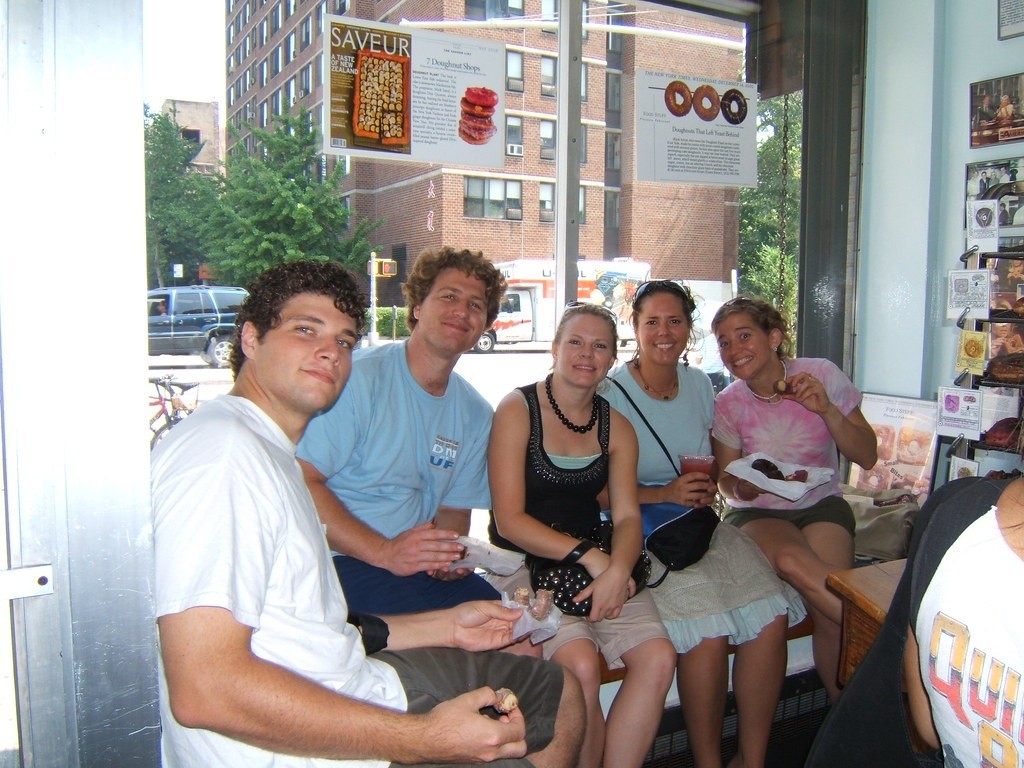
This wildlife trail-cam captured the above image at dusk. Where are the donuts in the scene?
[856,425,931,489]
[459,86,498,145]
[493,687,518,714]
[511,587,554,622]
[664,81,748,125]
[751,459,808,481]
[976,208,993,227]
[965,338,984,358]
[984,296,1024,446]
[773,380,797,395]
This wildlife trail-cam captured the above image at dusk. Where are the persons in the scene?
[158,300,166,316]
[710,297,878,702]
[801,473,1024,768]
[295,247,542,661]
[487,302,677,768]
[149,259,588,768]
[975,94,1024,359]
[596,280,808,768]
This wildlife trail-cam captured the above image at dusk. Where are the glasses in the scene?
[720,296,752,308]
[632,280,689,303]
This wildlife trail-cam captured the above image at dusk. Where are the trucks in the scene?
[471,257,652,355]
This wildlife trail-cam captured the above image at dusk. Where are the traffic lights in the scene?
[377,260,397,278]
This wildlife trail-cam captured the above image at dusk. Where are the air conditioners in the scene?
[508,143,524,158]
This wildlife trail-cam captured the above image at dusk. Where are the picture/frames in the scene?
[964,71,1024,458]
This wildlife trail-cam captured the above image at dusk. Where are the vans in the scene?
[147,284,256,369]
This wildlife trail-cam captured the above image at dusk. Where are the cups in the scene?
[679,453,715,503]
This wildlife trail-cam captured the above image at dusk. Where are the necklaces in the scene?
[751,360,787,399]
[546,374,598,433]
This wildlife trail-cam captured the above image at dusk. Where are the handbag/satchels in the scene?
[530,520,652,615]
[803,629,922,768]
[640,502,720,588]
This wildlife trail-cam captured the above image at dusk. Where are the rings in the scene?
[808,373,811,379]
